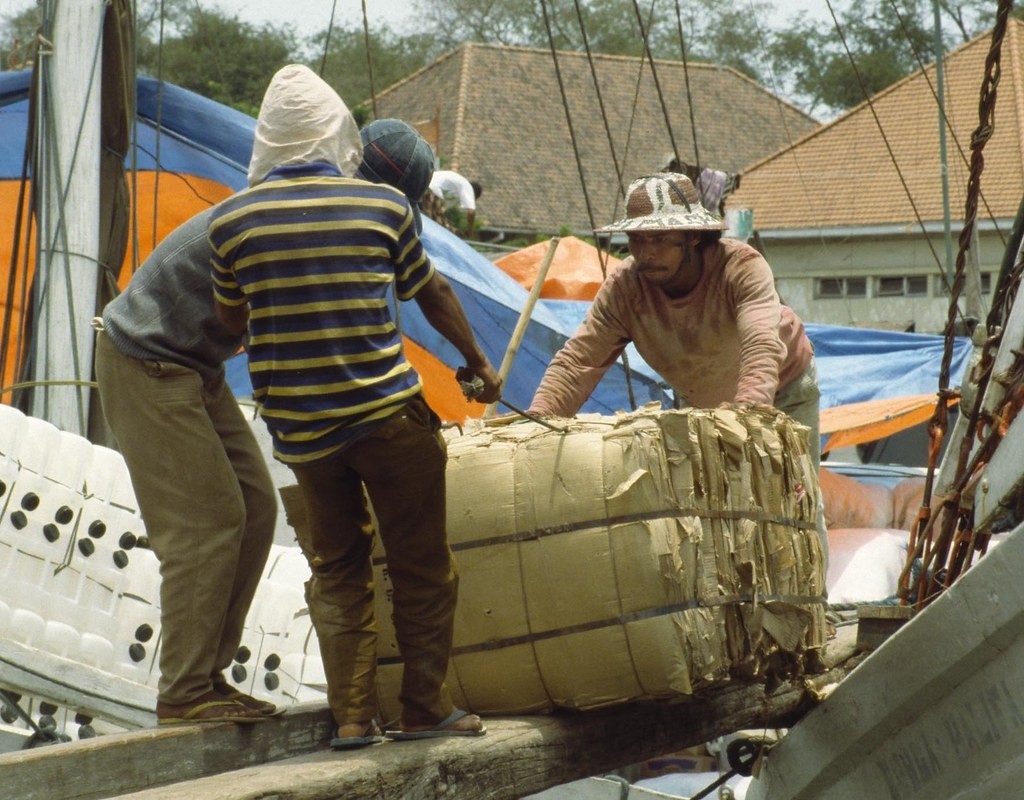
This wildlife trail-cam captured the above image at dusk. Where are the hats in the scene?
[357,118,434,207]
[593,172,726,233]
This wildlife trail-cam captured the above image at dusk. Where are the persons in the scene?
[528,173,820,475]
[92,118,435,723]
[428,170,482,238]
[206,63,504,747]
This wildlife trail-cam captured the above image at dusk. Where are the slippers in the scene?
[330,719,383,747]
[385,709,486,738]
[217,683,286,717]
[157,698,268,724]
